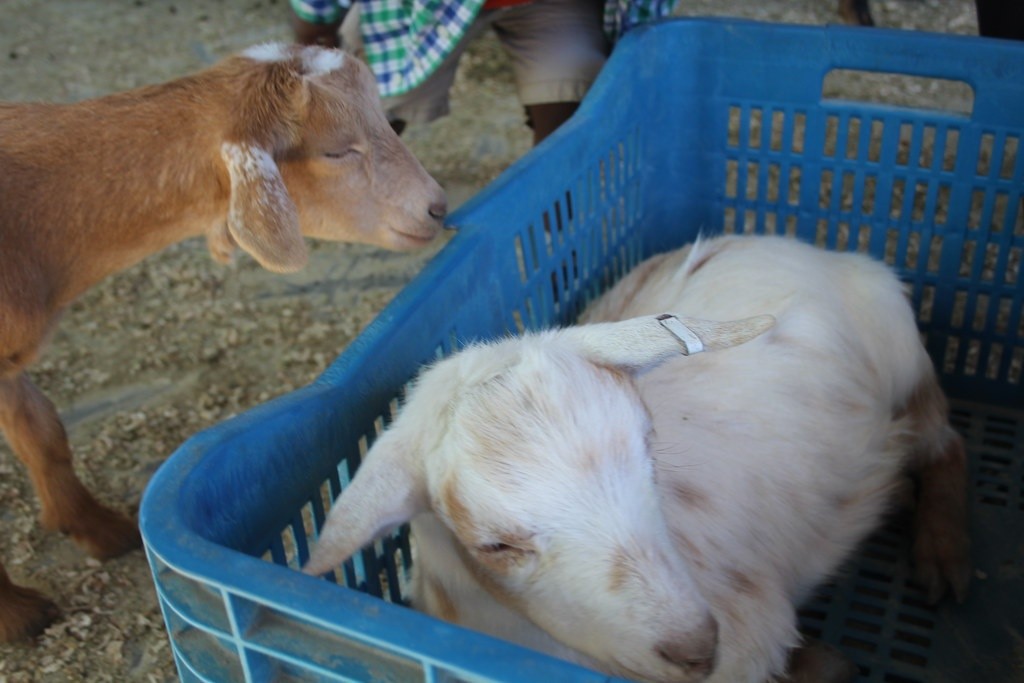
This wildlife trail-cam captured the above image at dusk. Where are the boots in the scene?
[524,101,582,230]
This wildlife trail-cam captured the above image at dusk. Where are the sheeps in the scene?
[0,0,449,646]
[303,234,961,683]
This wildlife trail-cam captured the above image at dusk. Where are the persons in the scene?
[279,0,671,312]
